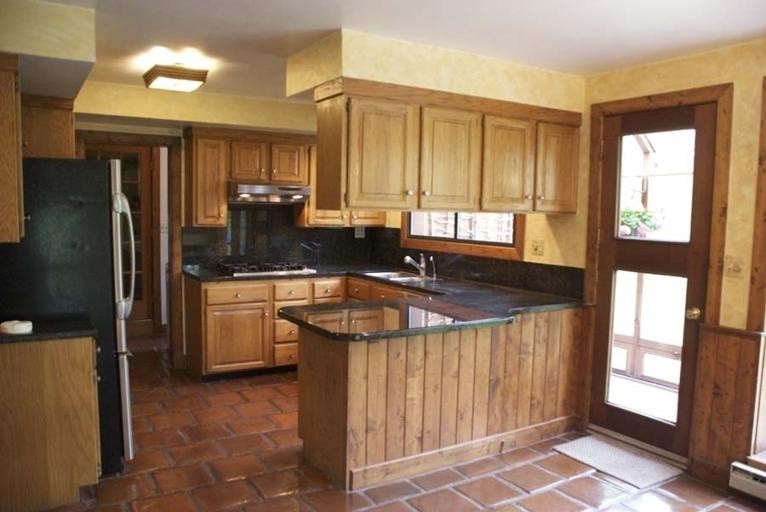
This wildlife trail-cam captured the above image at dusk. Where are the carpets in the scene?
[552,433,686,489]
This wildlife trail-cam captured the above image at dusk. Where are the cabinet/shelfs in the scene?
[482,114,580,214]
[293,144,386,228]
[185,276,416,376]
[230,139,309,186]
[317,95,483,210]
[1,315,101,512]
[185,137,229,228]
[1,51,32,242]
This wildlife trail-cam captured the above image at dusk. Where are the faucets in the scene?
[429,255,437,280]
[404,253,426,276]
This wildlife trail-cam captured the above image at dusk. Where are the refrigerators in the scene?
[1,156,137,479]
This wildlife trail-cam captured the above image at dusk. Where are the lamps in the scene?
[142,65,209,93]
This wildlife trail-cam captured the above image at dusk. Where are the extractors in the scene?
[227,181,312,213]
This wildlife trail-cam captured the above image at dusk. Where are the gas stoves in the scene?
[206,252,318,278]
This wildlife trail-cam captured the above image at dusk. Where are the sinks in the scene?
[364,271,446,282]
